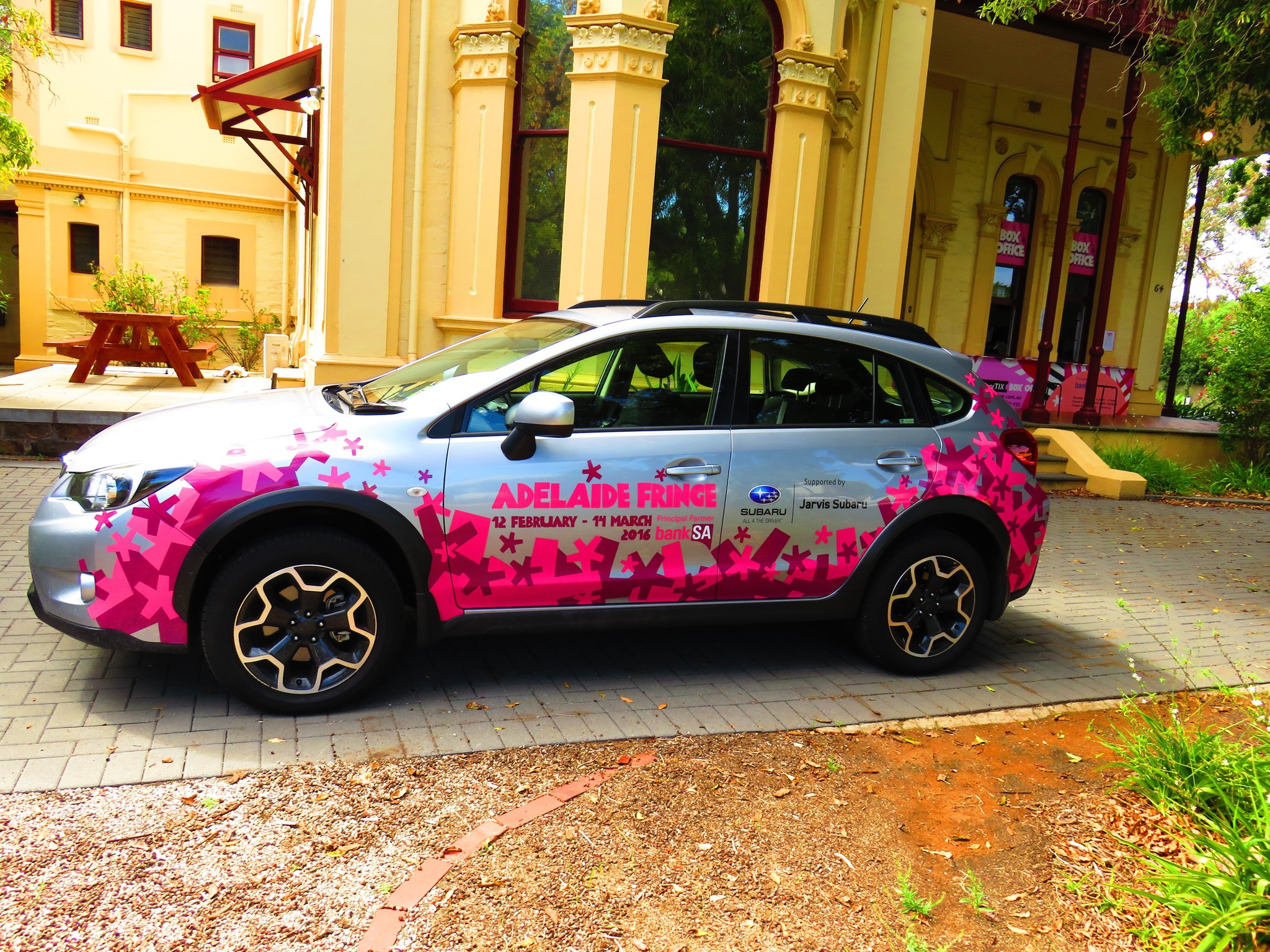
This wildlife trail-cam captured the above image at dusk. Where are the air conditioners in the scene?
[263,334,289,378]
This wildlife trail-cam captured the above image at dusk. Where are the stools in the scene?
[1097,385,1117,418]
[1030,383,1062,417]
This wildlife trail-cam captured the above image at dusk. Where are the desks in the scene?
[69,311,203,387]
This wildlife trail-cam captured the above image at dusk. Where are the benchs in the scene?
[758,368,851,423]
[42,336,218,360]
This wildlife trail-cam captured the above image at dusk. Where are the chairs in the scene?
[614,343,684,427]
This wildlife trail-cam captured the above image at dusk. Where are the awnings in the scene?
[191,44,320,231]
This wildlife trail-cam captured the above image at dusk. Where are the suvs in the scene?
[28,296,1050,713]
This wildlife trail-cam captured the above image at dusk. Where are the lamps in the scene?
[301,85,324,115]
[73,194,85,206]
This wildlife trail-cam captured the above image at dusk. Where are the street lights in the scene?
[1162,128,1220,422]
